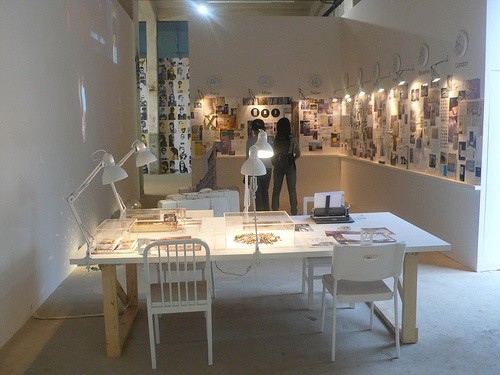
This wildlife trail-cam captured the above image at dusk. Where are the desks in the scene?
[70,212,452,359]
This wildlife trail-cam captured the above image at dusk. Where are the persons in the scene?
[138,59,190,175]
[246,119,273,211]
[271,118,301,215]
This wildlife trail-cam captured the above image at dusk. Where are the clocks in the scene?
[310,76,322,89]
[391,55,401,72]
[416,44,428,67]
[455,32,467,55]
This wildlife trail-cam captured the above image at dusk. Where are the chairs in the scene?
[143,210,215,368]
[304,197,406,361]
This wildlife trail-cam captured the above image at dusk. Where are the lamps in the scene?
[111,140,158,219]
[242,129,275,220]
[63,149,128,272]
[241,147,267,266]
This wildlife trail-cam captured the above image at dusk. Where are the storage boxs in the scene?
[94,208,163,246]
[224,211,295,232]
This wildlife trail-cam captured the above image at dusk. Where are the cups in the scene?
[360,230,374,246]
